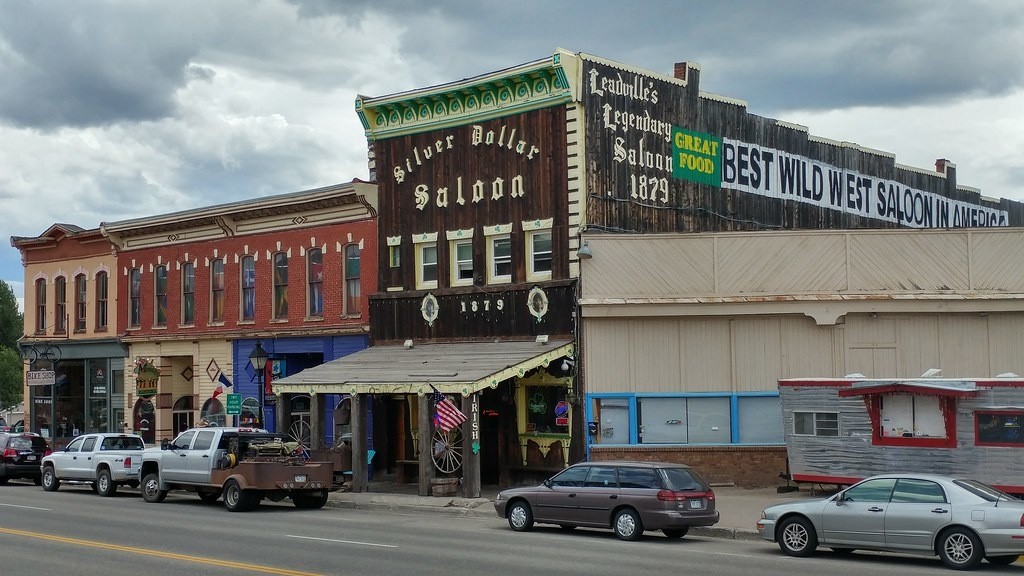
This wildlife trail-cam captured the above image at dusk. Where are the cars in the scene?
[494,462,720,541]
[14,420,24,433]
[0,416,10,433]
[757,474,1024,570]
[43,374,71,395]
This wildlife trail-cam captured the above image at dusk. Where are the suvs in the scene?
[0,432,53,486]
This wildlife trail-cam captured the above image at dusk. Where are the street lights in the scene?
[248,340,269,429]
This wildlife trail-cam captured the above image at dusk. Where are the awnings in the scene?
[270,341,575,398]
[837,380,985,401]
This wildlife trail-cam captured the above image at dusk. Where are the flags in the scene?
[430,382,469,434]
[212,370,234,400]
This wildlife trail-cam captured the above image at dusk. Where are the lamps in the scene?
[403,339,414,349]
[535,335,549,345]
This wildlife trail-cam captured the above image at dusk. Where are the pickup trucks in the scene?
[137,427,335,512]
[40,433,146,497]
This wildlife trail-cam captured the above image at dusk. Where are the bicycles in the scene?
[20,339,61,365]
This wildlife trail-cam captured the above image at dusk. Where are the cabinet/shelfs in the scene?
[310,449,351,472]
